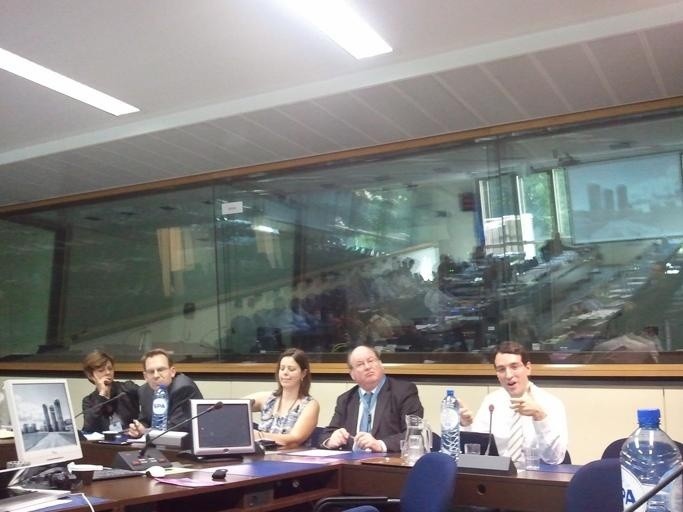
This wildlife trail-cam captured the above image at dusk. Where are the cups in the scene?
[523,446,542,472]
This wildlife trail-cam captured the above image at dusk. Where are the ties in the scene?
[509,411,524,461]
[359,395,371,432]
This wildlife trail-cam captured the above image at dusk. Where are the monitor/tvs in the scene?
[460,431,499,456]
[189,398,255,463]
[3,377,84,496]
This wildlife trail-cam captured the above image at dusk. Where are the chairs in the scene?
[430,432,441,452]
[601,438,682,463]
[311,427,325,448]
[565,458,624,511]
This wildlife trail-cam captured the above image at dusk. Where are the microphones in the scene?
[113,402,224,471]
[485,403,496,455]
[73,392,127,420]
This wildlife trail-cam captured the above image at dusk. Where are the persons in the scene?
[542,239,555,263]
[606,261,672,338]
[318,346,424,453]
[268,253,470,352]
[472,247,511,288]
[240,349,319,448]
[552,233,563,257]
[128,348,205,439]
[451,340,570,463]
[82,352,140,432]
[587,326,660,362]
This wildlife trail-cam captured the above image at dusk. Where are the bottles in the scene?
[151,384,169,433]
[618,407,683,512]
[440,388,460,462]
[401,414,433,467]
